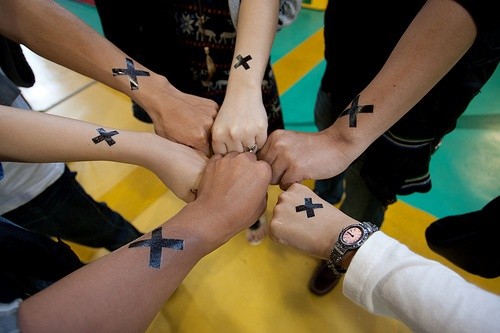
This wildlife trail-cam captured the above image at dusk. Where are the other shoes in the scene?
[312,189,342,205]
[396,179,431,196]
[308,259,342,295]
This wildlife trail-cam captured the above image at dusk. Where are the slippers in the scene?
[246,212,267,246]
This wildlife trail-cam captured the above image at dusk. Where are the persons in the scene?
[270,182,500,332]
[255,0,500,296]
[94,0,286,246]
[0,0,220,251]
[0,104,211,205]
[0,149,273,333]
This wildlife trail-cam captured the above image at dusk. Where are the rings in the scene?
[244,144,258,152]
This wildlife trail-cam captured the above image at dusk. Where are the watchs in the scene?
[327,220,379,276]
